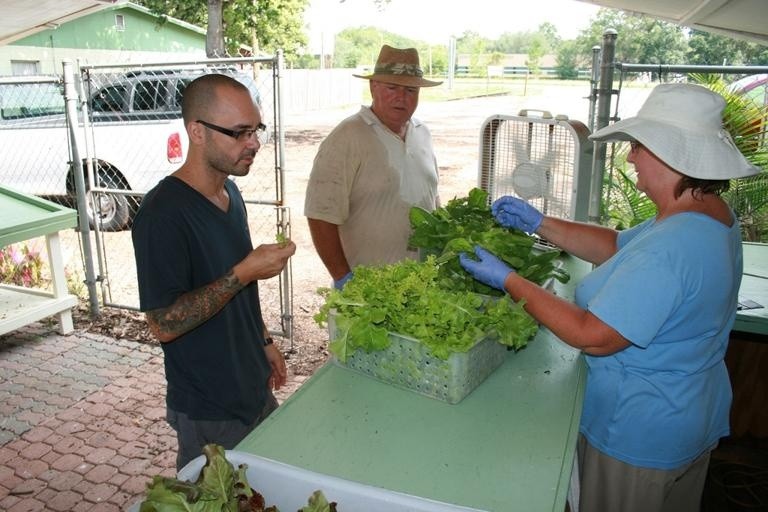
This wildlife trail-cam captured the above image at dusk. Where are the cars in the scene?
[723,72,768,151]
[87,67,271,146]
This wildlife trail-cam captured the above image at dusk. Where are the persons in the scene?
[457,82,761,510]
[129,75,290,468]
[303,47,448,290]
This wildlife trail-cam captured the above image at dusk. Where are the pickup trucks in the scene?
[0,74,189,232]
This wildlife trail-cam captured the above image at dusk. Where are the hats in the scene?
[352,44,444,88]
[587,83,762,180]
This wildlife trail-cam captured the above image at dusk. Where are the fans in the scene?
[476,108,593,224]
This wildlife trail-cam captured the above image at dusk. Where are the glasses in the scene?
[196,120,266,142]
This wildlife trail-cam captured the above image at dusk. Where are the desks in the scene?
[233,240,768,512]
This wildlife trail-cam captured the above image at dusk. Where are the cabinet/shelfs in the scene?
[0,185,79,335]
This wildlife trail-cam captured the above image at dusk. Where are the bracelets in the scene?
[263,337,273,346]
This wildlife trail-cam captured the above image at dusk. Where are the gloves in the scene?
[492,195,544,236]
[459,248,516,292]
